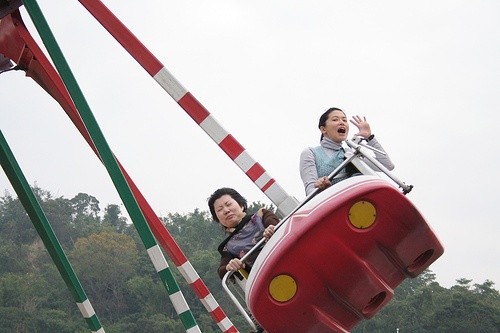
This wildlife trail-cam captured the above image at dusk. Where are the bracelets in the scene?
[365,134,374,142]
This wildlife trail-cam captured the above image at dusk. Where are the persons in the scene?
[300,107,395,197]
[207,188,280,284]
